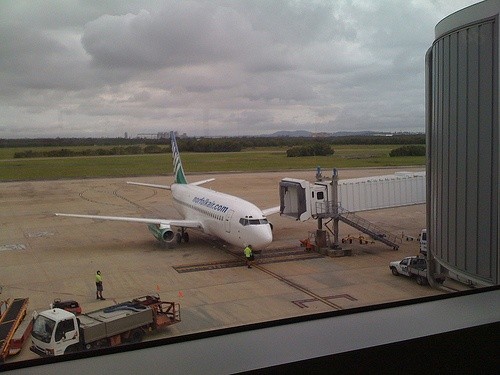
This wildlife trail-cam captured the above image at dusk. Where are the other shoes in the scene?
[100,298,105,300]
[97,297,100,299]
[248,267,252,268]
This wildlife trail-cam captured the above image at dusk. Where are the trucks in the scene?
[29,293,181,359]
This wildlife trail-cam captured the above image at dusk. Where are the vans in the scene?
[419,229,427,256]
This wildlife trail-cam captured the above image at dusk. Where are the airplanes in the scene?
[55,129,281,262]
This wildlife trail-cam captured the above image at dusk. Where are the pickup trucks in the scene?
[389,255,446,286]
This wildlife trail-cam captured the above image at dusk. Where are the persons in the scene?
[96,270,106,299]
[244,244,253,268]
